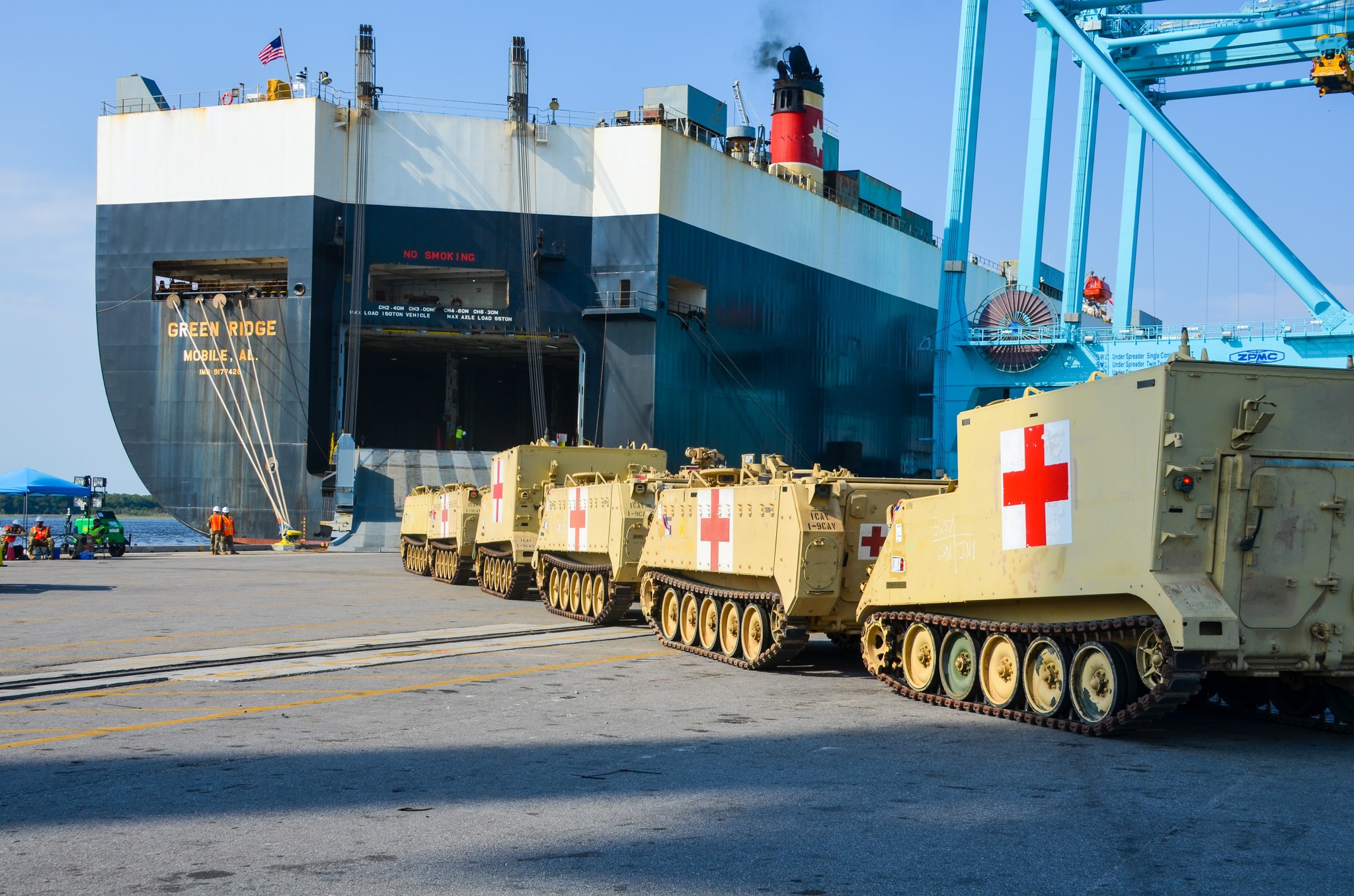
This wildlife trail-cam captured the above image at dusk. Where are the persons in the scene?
[28,516,55,560]
[72,518,106,560]
[207,506,225,555]
[220,507,239,555]
[2,519,26,559]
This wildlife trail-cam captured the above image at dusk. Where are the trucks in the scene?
[65,476,131,556]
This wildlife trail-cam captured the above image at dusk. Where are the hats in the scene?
[93,518,102,525]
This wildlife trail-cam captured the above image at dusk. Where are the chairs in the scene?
[34,544,47,559]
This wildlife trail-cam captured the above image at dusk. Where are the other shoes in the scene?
[230,551,239,554]
[222,551,228,554]
[0,565,8,567]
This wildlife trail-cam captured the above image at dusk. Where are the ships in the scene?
[93,21,1152,548]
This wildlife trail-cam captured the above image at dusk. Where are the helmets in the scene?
[222,507,229,513]
[212,506,221,512]
[36,516,44,521]
[13,519,21,525]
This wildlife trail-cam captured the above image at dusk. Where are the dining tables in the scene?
[86,531,107,559]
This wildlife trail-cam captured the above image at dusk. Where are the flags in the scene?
[258,35,284,68]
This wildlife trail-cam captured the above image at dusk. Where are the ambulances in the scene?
[400,326,1354,737]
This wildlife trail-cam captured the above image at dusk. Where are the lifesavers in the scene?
[450,298,461,306]
[221,93,233,105]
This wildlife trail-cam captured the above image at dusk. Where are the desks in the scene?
[1,534,76,542]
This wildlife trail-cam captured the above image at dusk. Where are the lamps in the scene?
[321,71,333,85]
[549,97,560,111]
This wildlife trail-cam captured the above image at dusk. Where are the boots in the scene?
[48,551,53,560]
[214,549,220,555]
[212,550,215,555]
[29,552,36,560]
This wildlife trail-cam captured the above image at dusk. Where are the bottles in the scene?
[2,527,5,534]
[0,527,3,534]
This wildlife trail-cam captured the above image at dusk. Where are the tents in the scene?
[0,463,91,548]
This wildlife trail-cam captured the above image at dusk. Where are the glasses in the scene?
[93,523,96,524]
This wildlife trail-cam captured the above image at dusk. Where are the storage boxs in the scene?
[78,551,94,560]
